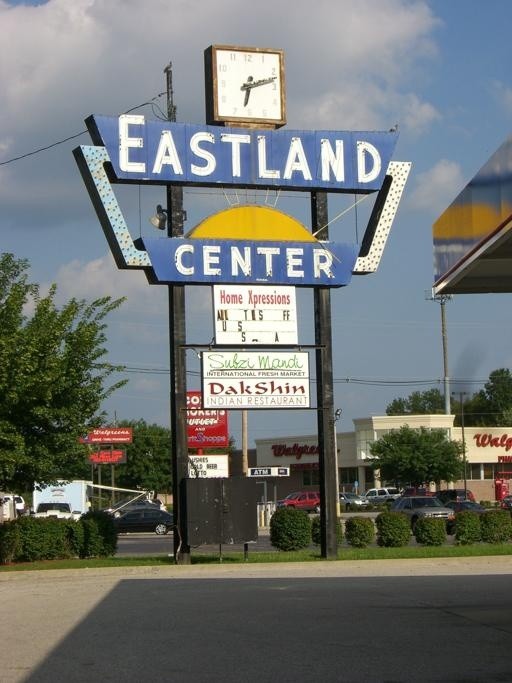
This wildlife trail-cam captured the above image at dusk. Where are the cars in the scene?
[500,495,512,510]
[278,485,485,534]
[3,477,175,536]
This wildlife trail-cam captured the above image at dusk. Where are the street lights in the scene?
[450,388,467,501]
[148,204,193,566]
[333,407,345,520]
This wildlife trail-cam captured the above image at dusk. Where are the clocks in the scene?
[204,44,287,128]
